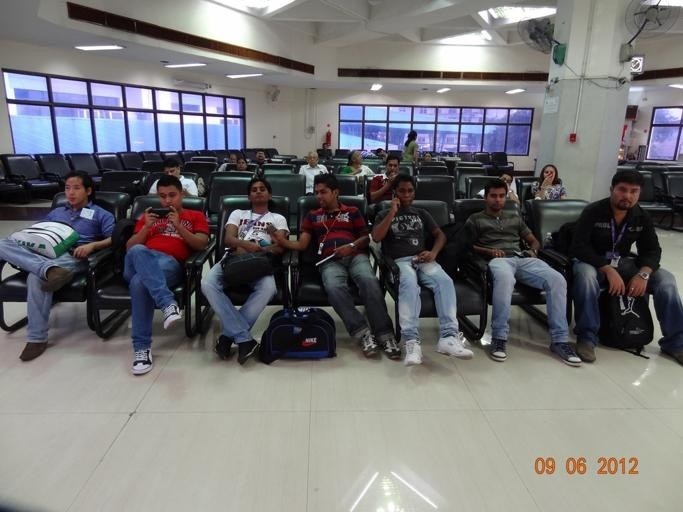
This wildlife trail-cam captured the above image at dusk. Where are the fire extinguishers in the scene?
[326,130,331,146]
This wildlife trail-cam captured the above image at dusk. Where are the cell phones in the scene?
[392,189,397,197]
[150,208,169,218]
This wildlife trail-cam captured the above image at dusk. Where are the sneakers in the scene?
[661,346,683,364]
[132,349,153,375]
[550,336,596,367]
[161,304,182,329]
[490,339,507,361]
[216,334,259,364]
[435,332,474,360]
[362,329,423,365]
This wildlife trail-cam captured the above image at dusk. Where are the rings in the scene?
[628,287,633,291]
[495,254,498,255]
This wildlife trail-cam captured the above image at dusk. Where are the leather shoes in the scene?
[20,342,48,361]
[42,268,66,291]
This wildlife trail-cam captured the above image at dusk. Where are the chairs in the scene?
[0,148,683,339]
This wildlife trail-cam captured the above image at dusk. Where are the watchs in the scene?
[530,248,537,254]
[347,242,357,253]
[636,269,649,281]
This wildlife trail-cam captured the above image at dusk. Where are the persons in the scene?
[569,170,682,365]
[371,173,474,365]
[0,170,115,360]
[424,152,433,161]
[299,151,329,196]
[123,175,209,375]
[200,179,289,363]
[149,158,199,196]
[466,180,581,367]
[274,173,400,359]
[479,171,518,201]
[531,164,567,200]
[342,148,400,198]
[218,151,265,174]
[403,131,418,162]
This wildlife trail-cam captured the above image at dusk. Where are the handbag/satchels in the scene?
[258,307,336,365]
[222,252,273,280]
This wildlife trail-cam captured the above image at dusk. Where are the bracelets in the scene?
[508,189,513,193]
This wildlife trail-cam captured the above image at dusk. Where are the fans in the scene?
[518,16,565,63]
[620,0,680,62]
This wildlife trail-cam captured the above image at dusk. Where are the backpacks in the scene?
[599,289,653,359]
